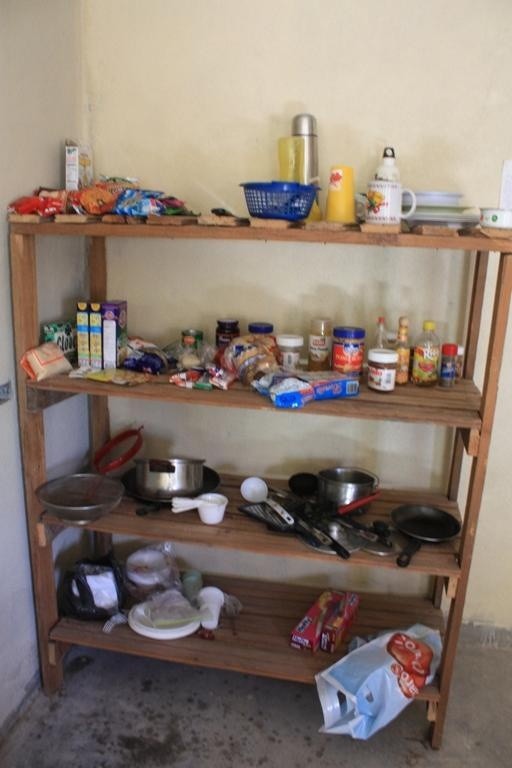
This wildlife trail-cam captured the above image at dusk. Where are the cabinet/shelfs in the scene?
[8,215,512,751]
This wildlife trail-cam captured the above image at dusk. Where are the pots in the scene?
[319,467,374,516]
[391,501,460,567]
[122,454,220,516]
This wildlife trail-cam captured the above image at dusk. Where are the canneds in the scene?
[331,326,365,381]
[181,328,204,351]
[367,348,399,393]
[216,319,241,349]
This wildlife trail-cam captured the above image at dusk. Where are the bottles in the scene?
[375,147,400,182]
[373,316,459,390]
[292,112,320,187]
[307,318,332,371]
[324,164,357,225]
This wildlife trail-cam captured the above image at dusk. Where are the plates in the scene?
[402,191,479,228]
[129,601,200,641]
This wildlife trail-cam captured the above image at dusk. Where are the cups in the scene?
[367,180,415,226]
[278,138,303,182]
[197,586,225,631]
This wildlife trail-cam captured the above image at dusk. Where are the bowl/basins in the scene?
[127,550,170,586]
[482,207,512,229]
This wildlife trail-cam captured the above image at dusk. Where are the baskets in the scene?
[240,182,319,220]
